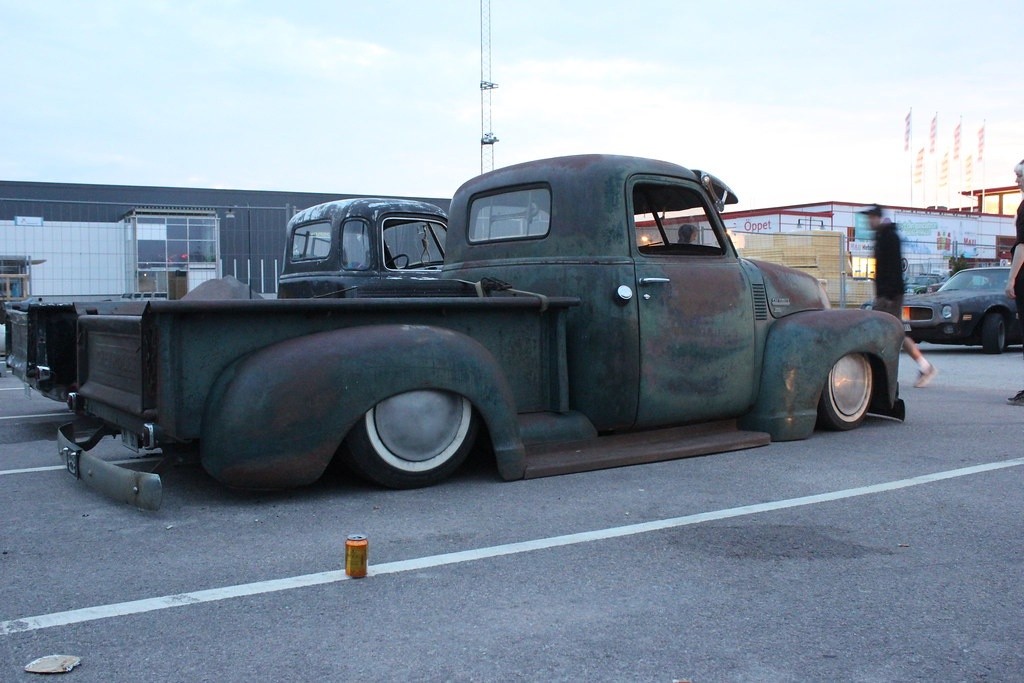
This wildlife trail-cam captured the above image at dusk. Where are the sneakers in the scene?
[1007,390,1024,406]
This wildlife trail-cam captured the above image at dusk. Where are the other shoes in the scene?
[913,363,937,388]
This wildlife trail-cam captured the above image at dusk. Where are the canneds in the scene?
[345,534,368,578]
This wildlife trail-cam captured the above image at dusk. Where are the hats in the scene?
[860,204,881,217]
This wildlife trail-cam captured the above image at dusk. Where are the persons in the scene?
[1007,160,1024,405]
[678,224,698,243]
[860,206,938,388]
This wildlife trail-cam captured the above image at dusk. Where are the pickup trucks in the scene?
[0,197,454,405]
[53,153,909,510]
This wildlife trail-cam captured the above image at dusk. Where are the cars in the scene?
[902,265,1024,355]
[861,271,948,311]
